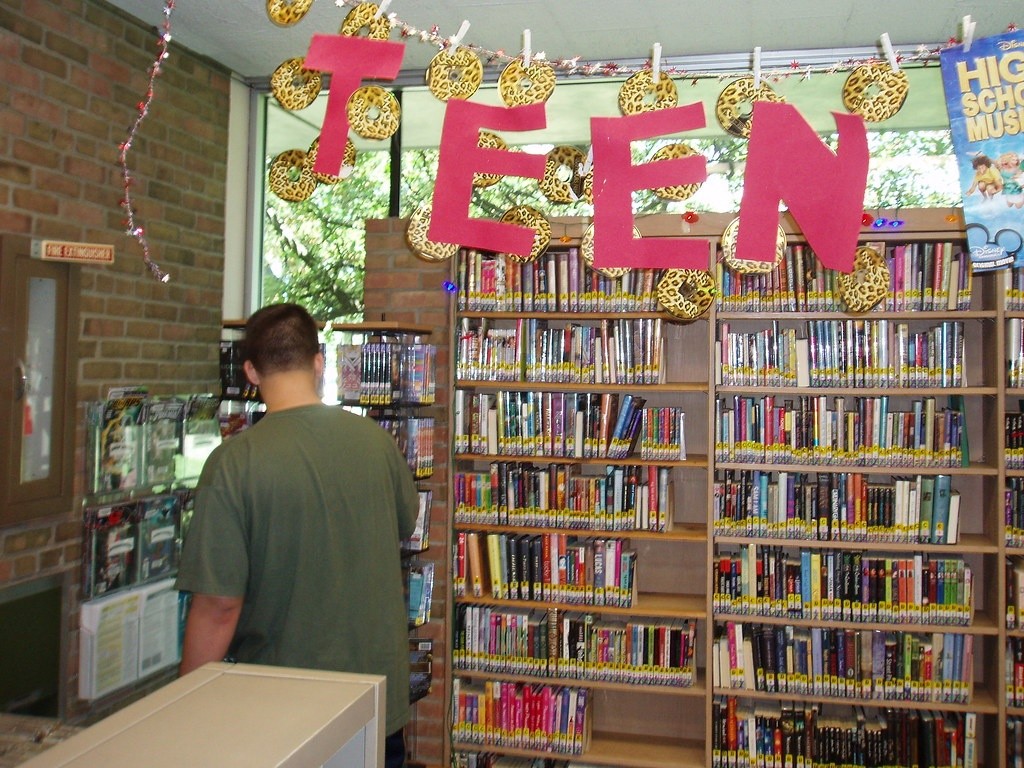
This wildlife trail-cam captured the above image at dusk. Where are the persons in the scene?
[172,301,422,768]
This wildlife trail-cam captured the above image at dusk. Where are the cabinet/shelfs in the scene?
[332,322,434,706]
[444,208,1024,768]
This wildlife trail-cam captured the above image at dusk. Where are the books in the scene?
[76,240,1024,768]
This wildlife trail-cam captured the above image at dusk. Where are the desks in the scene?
[18,661,387,768]
